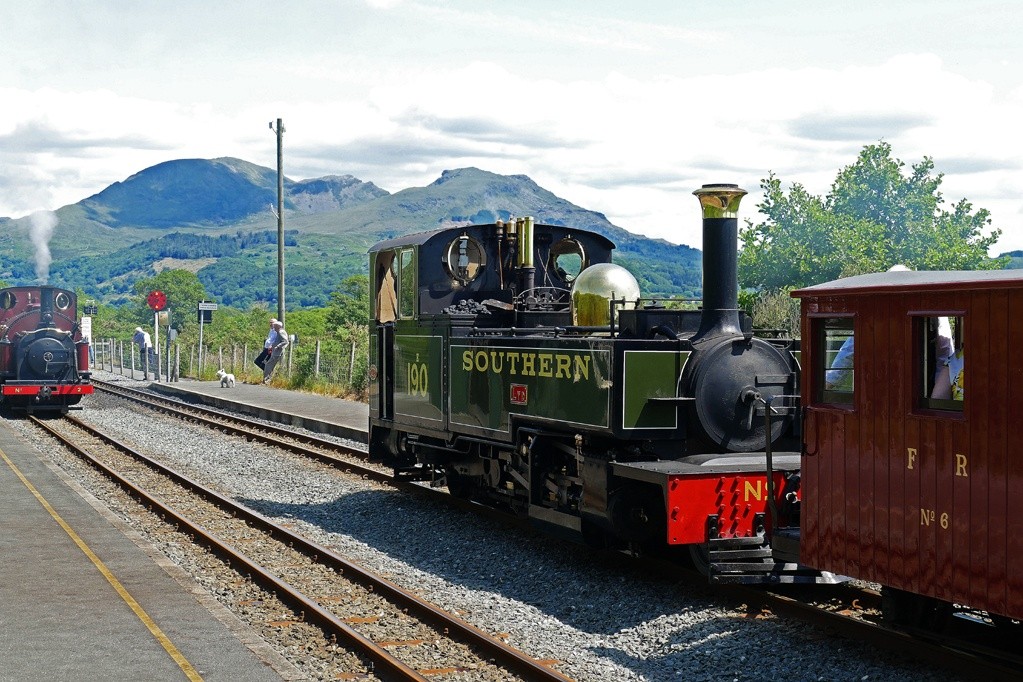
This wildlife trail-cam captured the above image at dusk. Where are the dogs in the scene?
[216,369,236,388]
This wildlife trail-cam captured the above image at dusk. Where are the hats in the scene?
[273,321,283,327]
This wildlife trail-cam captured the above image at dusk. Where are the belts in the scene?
[264,348,268,350]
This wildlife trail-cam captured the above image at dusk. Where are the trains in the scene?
[0,284,94,416]
[366,182,1023,639]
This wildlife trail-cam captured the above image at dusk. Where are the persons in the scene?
[132,327,159,380]
[88,345,94,362]
[253,319,278,370]
[826,263,955,393]
[264,321,289,384]
[931,350,964,401]
